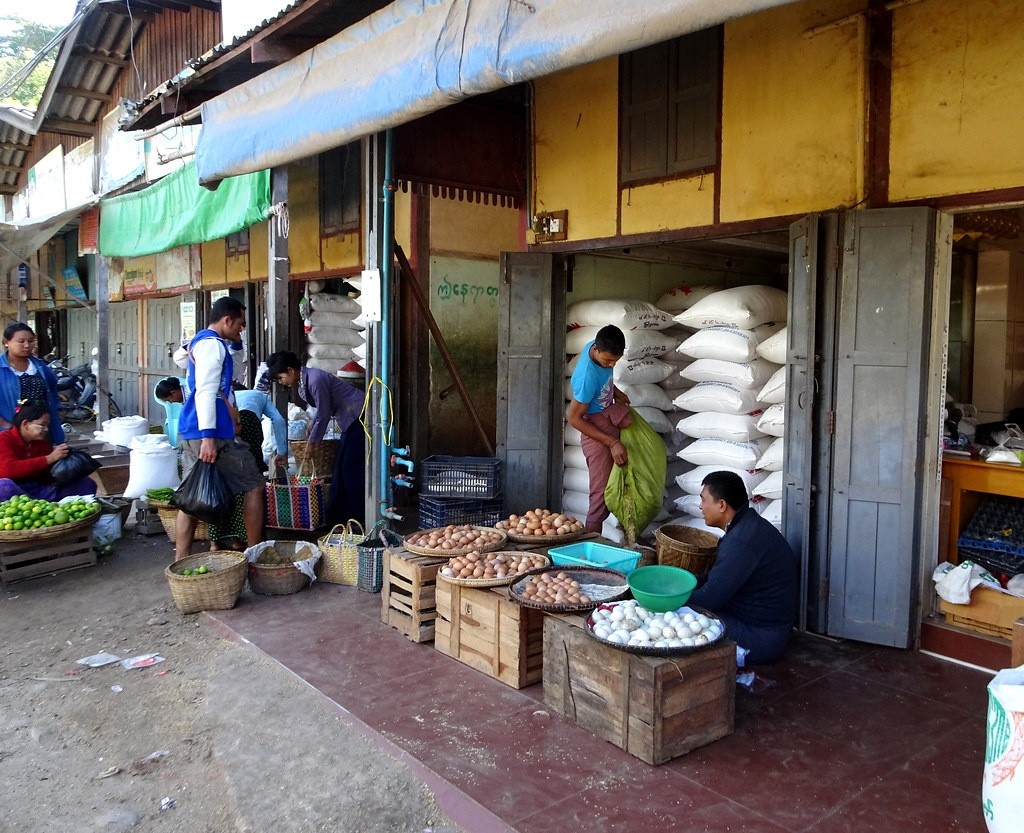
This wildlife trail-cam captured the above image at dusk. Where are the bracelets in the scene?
[611,439,619,448]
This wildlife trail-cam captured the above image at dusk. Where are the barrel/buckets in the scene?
[99,512,123,539]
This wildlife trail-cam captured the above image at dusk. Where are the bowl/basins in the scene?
[627,565,698,613]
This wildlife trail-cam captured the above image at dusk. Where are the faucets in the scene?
[385,506,404,522]
[395,474,415,488]
[390,454,414,473]
[400,445,410,457]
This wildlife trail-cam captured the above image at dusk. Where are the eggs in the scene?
[406,524,502,550]
[521,572,591,604]
[591,600,720,647]
[495,508,583,535]
[440,550,545,580]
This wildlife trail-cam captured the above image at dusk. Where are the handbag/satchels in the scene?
[264,454,325,530]
[51,447,103,484]
[356,520,405,594]
[168,442,237,529]
[317,519,370,586]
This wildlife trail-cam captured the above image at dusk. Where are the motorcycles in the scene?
[46,353,122,426]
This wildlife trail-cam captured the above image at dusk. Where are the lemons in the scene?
[181,565,209,576]
[0,494,100,532]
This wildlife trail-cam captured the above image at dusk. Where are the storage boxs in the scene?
[938,586,1024,641]
[380,454,739,766]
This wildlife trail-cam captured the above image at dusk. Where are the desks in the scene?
[933,453,1024,564]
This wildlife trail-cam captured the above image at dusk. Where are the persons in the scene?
[266,351,366,533]
[566,325,631,535]
[207,382,287,550]
[0,322,65,446]
[0,398,72,501]
[155,377,191,404]
[174,297,267,560]
[686,471,798,695]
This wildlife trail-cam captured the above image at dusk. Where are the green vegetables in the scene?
[145,487,175,502]
